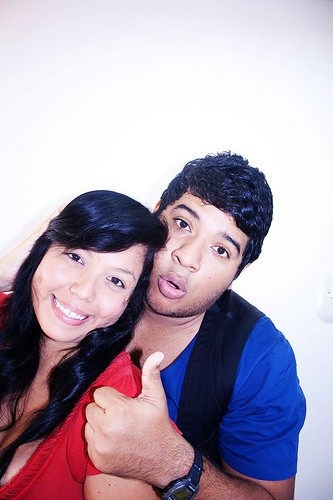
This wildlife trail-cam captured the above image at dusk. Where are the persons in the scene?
[0,190,183,500]
[0,150,307,500]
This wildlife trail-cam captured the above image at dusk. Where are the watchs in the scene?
[153,448,203,500]
